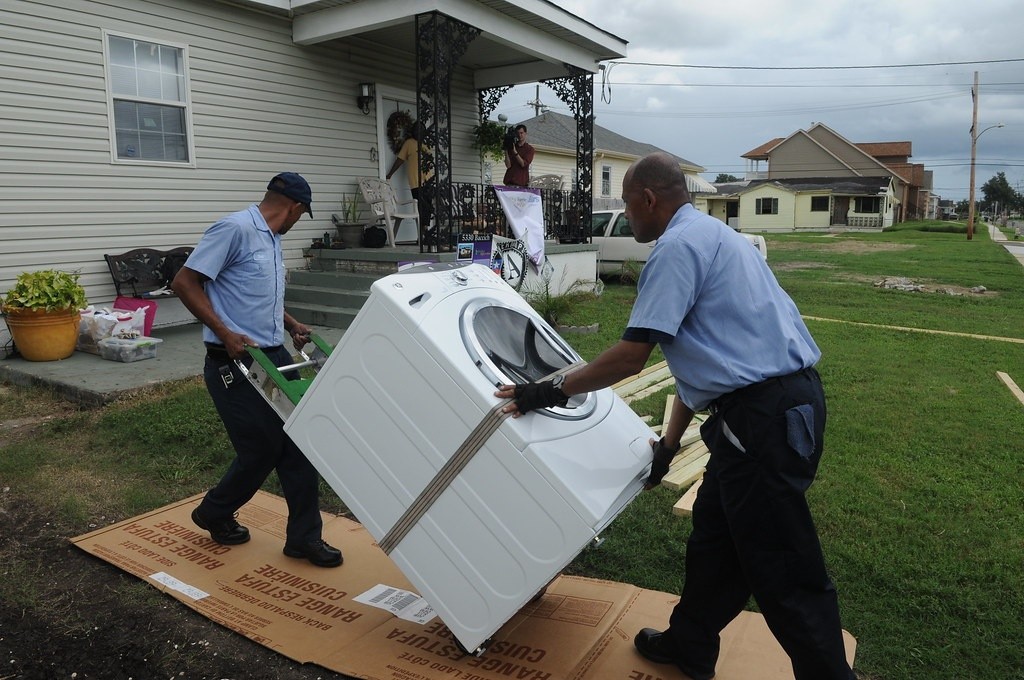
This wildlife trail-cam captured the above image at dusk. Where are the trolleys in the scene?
[225,333,494,657]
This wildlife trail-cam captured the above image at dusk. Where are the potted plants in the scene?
[421,228,438,252]
[0,267,89,362]
[333,183,368,247]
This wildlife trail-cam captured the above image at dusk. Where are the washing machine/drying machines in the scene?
[282,260,663,654]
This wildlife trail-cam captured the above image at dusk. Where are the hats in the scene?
[268,173,313,219]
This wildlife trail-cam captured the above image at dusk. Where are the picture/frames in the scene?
[457,243,474,261]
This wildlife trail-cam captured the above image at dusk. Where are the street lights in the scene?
[968,124,1004,240]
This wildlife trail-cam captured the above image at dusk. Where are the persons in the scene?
[503,124,535,187]
[386,121,434,244]
[493,152,859,680]
[171,172,344,567]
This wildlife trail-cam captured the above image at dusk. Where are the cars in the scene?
[581,210,767,278]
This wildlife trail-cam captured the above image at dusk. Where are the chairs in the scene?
[527,174,564,238]
[359,177,420,249]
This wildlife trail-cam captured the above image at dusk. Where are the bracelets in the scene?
[514,152,519,156]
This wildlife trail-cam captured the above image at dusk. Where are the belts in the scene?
[707,368,810,416]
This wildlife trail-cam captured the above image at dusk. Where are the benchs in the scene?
[104,247,196,299]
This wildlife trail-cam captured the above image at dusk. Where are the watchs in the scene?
[551,375,570,407]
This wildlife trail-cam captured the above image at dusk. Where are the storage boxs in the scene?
[76,309,145,357]
[98,336,163,362]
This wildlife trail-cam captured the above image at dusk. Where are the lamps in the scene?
[356,81,374,115]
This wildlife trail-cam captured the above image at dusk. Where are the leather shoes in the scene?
[191,497,249,545]
[634,628,716,680]
[284,537,343,567]
